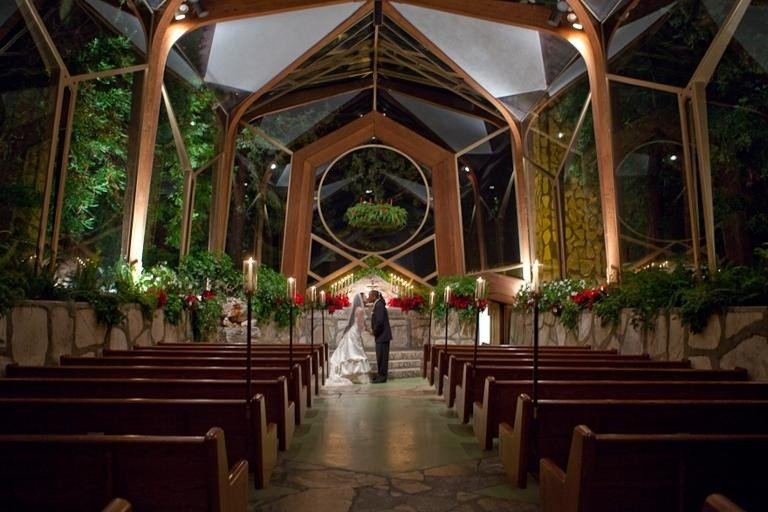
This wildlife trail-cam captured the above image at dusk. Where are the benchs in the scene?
[0,343,331,512]
[420,342,768,512]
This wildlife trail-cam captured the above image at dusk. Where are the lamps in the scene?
[547,8,562,27]
[192,3,209,18]
[175,10,185,20]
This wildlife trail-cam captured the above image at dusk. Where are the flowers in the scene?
[387,274,613,341]
[136,248,352,338]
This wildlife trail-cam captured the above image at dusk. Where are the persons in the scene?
[326,292,373,384]
[366,290,392,384]
